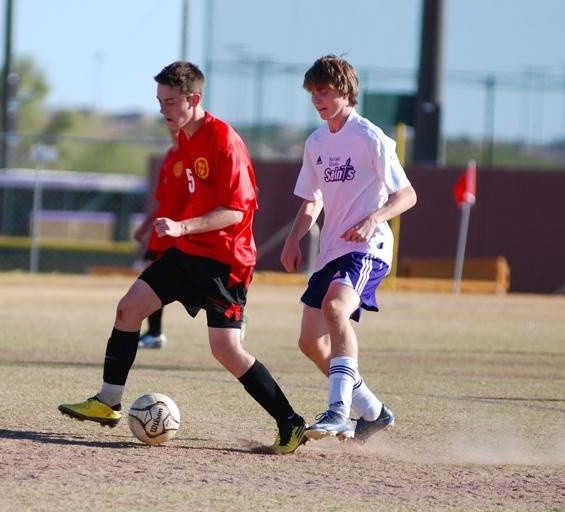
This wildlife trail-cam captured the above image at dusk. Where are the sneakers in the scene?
[138,334,163,348]
[59,397,122,428]
[272,404,395,455]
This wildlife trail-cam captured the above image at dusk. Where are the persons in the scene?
[279,54,417,443]
[131,130,245,349]
[57,60,309,455]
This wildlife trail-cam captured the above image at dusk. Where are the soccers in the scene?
[128,393,180,445]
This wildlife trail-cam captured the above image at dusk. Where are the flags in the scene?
[451,165,476,208]
